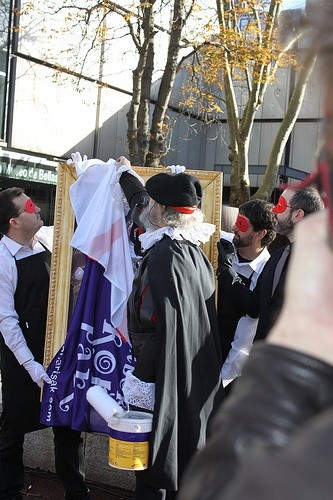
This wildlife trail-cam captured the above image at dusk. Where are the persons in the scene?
[0,152,92,500]
[177,211,333,500]
[216,185,325,398]
[115,156,226,500]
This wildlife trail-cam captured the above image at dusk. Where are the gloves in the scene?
[165,165,185,174]
[66,152,87,164]
[21,358,50,388]
[217,237,234,272]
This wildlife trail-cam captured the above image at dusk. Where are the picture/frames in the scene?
[40,163,224,403]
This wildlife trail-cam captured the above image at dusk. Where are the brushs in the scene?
[85,384,130,424]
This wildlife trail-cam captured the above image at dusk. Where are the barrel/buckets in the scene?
[108,403,153,470]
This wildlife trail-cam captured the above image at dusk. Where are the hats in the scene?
[145,172,201,208]
